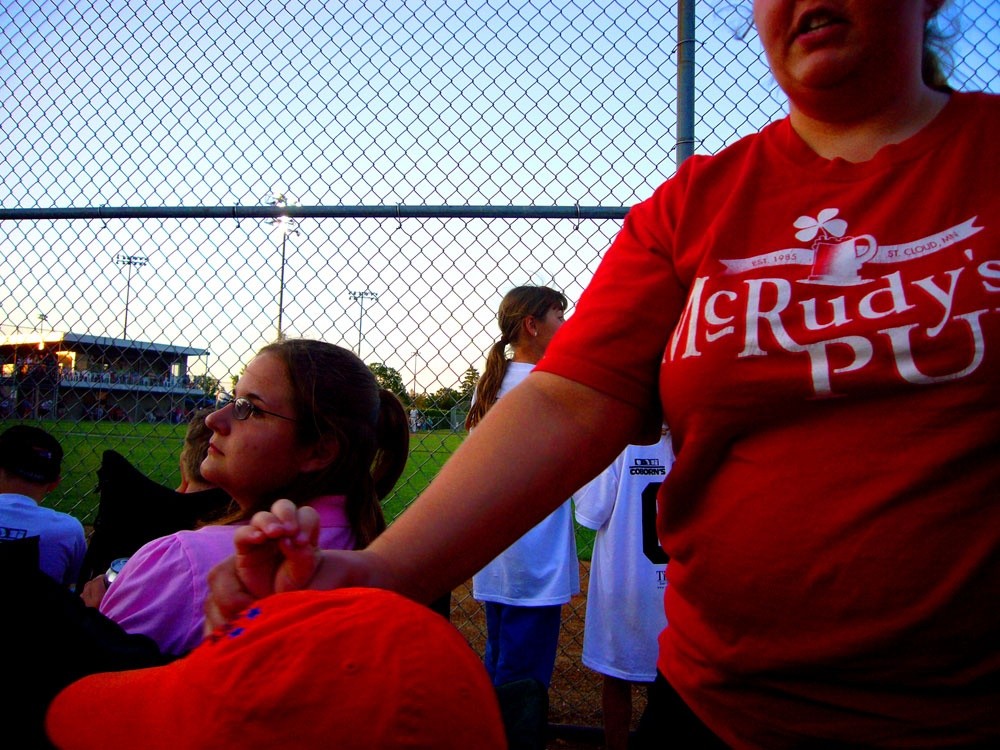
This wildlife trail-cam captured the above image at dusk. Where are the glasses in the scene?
[214,392,299,425]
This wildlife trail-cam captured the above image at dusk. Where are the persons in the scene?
[199,0,1000,749]
[462,284,580,690]
[0,338,509,750]
[569,417,678,750]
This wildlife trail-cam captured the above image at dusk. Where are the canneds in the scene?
[104,557,129,590]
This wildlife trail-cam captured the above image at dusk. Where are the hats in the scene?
[0,424,64,466]
[45,586,508,750]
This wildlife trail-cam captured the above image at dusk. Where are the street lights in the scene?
[264,192,300,344]
[411,352,421,407]
[349,291,379,358]
[39,313,48,332]
[116,254,148,340]
